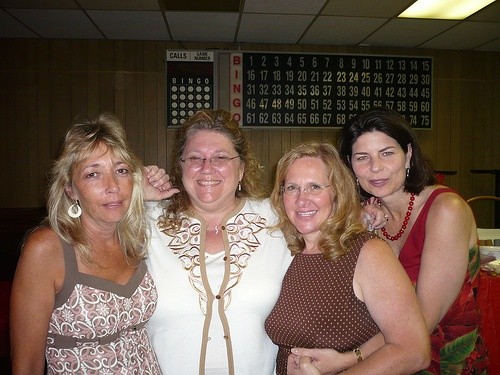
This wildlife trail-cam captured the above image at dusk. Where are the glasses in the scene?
[179,153,240,168]
[280,183,331,195]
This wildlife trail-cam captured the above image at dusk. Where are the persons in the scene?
[140,109,388,375]
[291,110,488,375]
[9,109,165,375]
[264,138,432,375]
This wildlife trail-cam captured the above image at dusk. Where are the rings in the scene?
[385,214,389,219]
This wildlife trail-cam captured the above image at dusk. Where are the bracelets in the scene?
[351,347,362,363]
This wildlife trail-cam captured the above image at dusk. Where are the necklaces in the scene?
[377,192,413,242]
[205,221,222,234]
